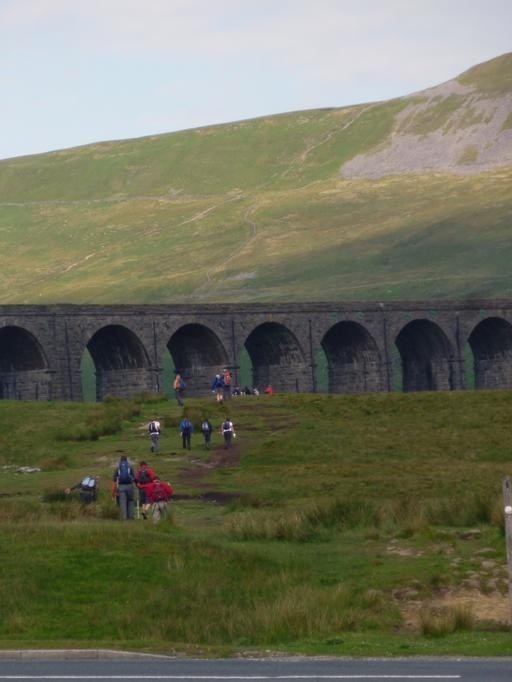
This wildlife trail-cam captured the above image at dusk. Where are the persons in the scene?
[64,473,102,504]
[199,416,213,449]
[146,475,173,525]
[135,460,156,520]
[211,368,273,404]
[173,374,187,407]
[146,417,162,454]
[221,416,235,450]
[111,477,120,510]
[111,454,139,519]
[179,414,193,451]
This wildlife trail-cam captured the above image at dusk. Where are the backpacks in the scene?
[117,460,132,484]
[201,420,210,432]
[223,371,232,385]
[223,422,231,430]
[138,469,151,484]
[179,380,185,392]
[149,422,157,433]
[216,379,223,388]
[79,477,96,490]
[151,483,169,502]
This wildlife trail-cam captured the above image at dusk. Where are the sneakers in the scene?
[141,510,147,522]
